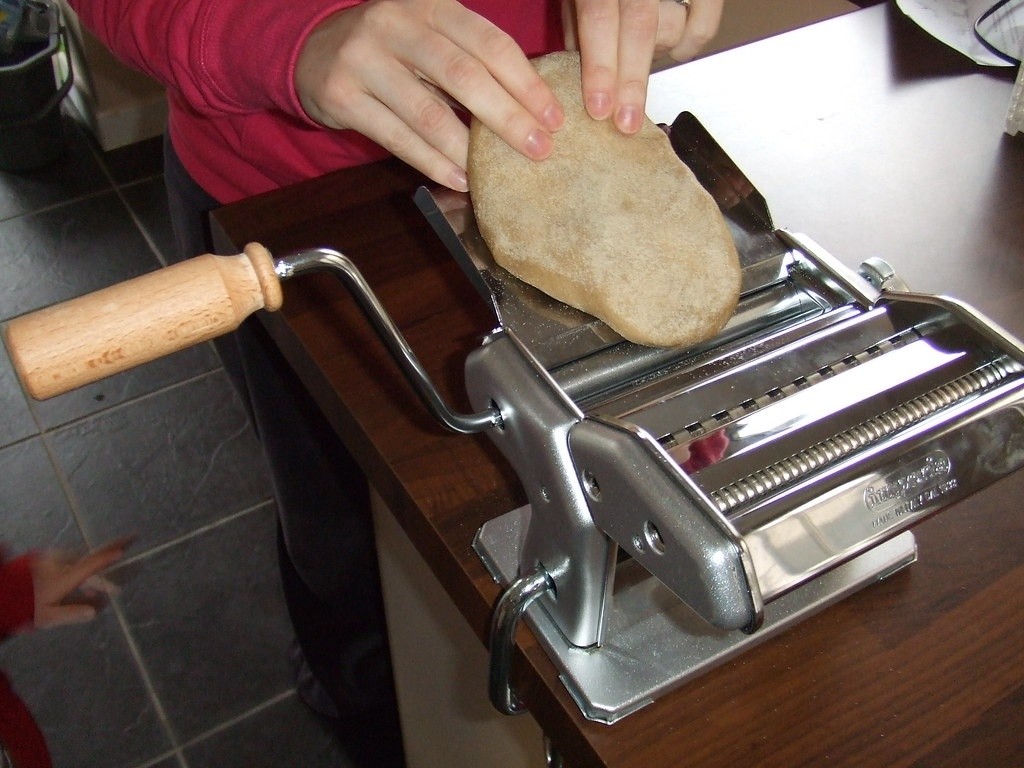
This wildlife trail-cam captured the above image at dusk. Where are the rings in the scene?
[675,0,691,8]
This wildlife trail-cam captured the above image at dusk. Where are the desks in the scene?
[211,0,1024,768]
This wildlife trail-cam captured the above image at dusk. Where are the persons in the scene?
[0,531,145,768]
[56,0,726,768]
